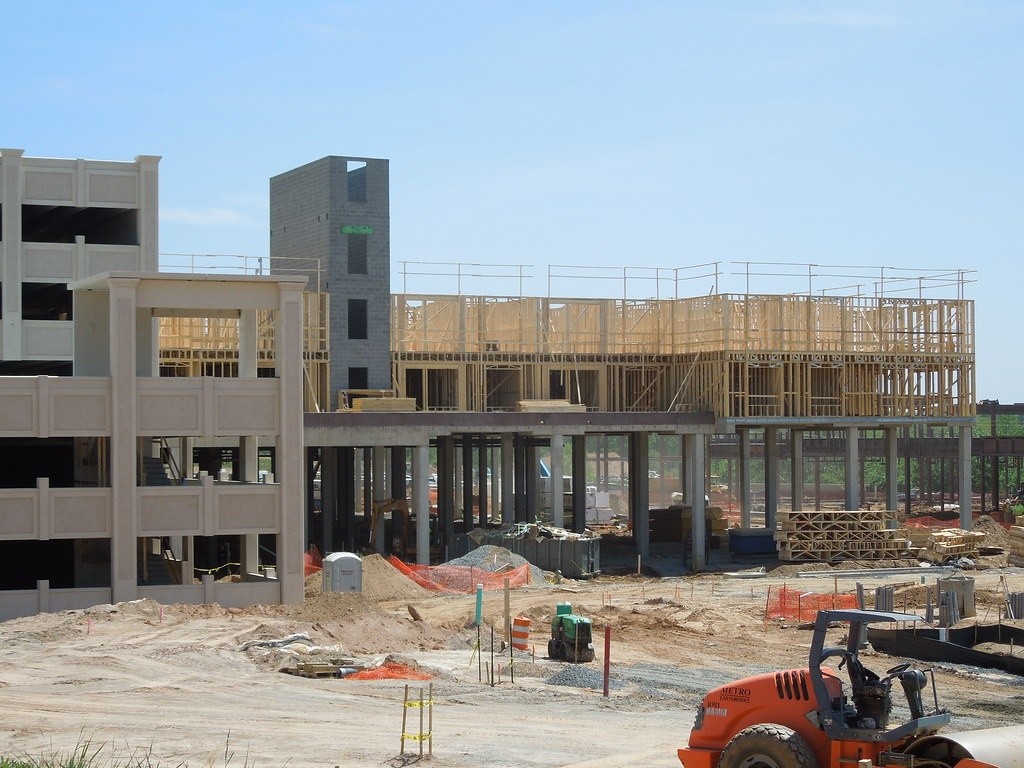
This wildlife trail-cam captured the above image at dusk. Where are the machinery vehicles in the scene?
[677,608,998,768]
[548,614,594,662]
[364,497,443,565]
